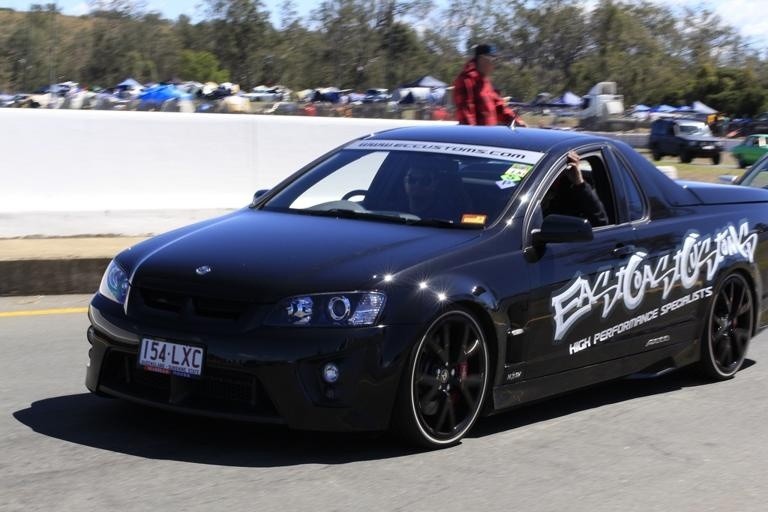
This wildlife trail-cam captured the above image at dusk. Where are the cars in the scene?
[732,151,768,189]
[1,77,458,121]
[730,133,768,170]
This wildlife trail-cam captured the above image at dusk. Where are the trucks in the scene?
[574,80,652,131]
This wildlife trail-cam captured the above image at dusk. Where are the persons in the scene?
[566,149,609,227]
[386,161,456,218]
[452,43,528,128]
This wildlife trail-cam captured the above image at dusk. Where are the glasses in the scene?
[406,174,437,185]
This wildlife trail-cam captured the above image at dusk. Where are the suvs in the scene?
[649,118,724,165]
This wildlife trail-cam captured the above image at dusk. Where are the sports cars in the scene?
[81,122,765,448]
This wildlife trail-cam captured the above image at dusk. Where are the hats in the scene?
[474,44,502,56]
